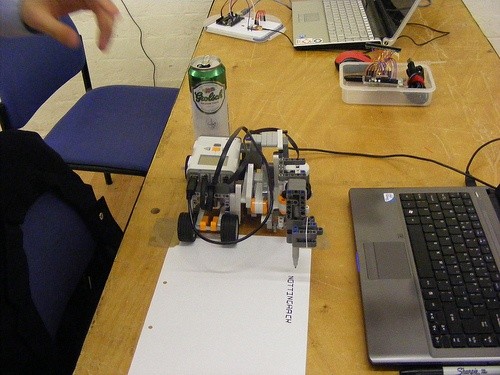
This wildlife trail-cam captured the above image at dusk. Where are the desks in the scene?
[72,0,500,375]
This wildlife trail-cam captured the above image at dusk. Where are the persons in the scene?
[0,0,119,51]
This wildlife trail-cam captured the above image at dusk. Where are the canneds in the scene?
[188,55,230,138]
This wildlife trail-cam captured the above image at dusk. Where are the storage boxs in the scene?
[339,62,437,106]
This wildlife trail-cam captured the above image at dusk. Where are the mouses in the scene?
[335,51,374,70]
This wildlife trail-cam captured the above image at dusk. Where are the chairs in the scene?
[0,0,181,186]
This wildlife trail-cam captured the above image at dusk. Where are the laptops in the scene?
[290,0,421,52]
[349,185,500,369]
